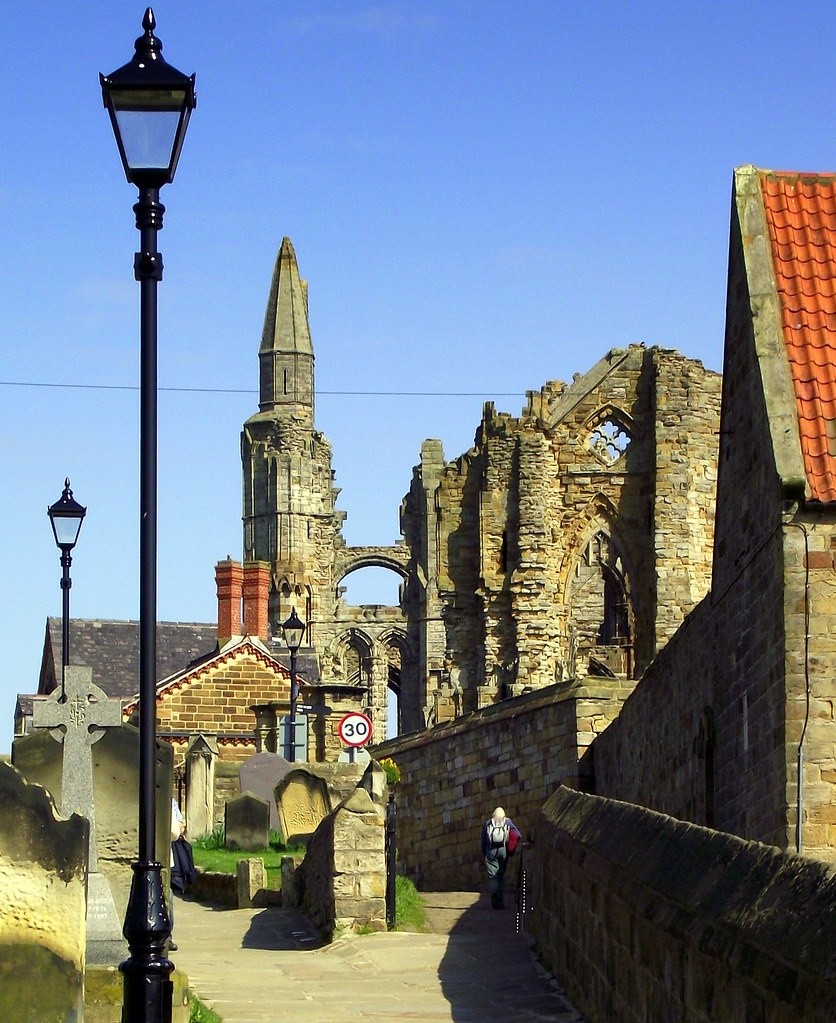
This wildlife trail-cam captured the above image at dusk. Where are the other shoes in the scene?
[168,940,178,950]
[491,892,505,910]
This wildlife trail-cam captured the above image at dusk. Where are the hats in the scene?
[492,806,505,816]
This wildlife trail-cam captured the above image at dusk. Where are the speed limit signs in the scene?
[339,715,373,748]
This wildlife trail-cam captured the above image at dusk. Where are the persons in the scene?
[171,797,187,952]
[481,807,523,909]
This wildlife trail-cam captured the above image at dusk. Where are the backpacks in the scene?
[486,817,510,848]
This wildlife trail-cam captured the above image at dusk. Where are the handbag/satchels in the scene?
[507,829,518,856]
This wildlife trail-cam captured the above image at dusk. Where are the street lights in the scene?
[47,479,87,705]
[96,0,198,1023]
[282,609,307,758]
